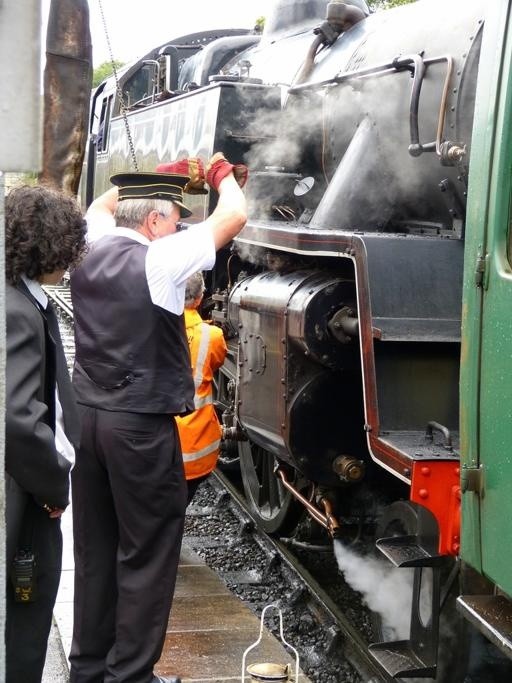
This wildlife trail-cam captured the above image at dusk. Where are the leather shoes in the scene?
[157,675,181,683]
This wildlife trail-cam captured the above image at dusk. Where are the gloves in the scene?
[204,151,249,192]
[157,157,208,195]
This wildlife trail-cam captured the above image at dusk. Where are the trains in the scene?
[77,0,511,677]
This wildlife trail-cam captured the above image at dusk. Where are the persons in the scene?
[174,271,229,508]
[5,182,92,590]
[68,149,251,683]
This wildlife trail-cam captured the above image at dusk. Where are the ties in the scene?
[46,298,83,451]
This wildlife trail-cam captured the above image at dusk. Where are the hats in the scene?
[109,171,191,218]
